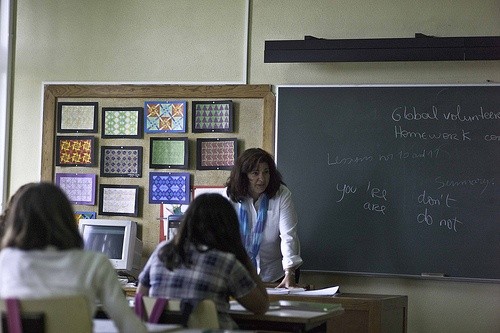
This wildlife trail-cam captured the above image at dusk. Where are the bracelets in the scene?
[284,271,295,275]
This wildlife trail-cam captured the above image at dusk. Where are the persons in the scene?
[0,182,148,333]
[137,193,269,333]
[226,148,304,290]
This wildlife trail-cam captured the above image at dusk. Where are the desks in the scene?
[94,282,409,333]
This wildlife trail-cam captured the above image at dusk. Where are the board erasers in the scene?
[421,272,444,277]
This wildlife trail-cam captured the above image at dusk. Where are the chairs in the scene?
[0,293,93,333]
[135,293,220,333]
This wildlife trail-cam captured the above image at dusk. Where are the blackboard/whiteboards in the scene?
[273,85,500,284]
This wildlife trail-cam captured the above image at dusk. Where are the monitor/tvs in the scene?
[78,219,143,273]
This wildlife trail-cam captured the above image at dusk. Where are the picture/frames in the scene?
[98,184,139,217]
[57,102,98,133]
[55,136,96,167]
[101,107,142,139]
[149,137,188,169]
[56,172,96,205]
[144,100,186,133]
[149,172,190,205]
[192,100,234,133]
[72,211,96,226]
[100,146,143,178]
[196,137,237,170]
